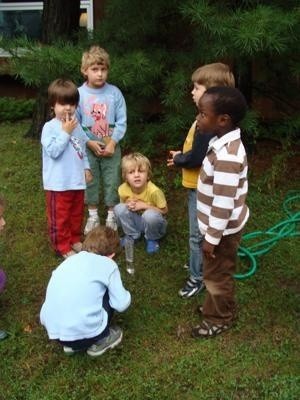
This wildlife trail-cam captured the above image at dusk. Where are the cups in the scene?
[125,240,134,274]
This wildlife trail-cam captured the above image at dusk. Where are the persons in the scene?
[191,86,250,338]
[75,44,129,235]
[168,61,239,297]
[42,79,93,258]
[1,200,8,296]
[40,227,134,358]
[111,152,168,256]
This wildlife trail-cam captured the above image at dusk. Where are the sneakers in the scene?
[194,304,207,316]
[182,262,192,272]
[119,236,140,248]
[0,330,10,341]
[176,274,206,300]
[83,213,101,236]
[105,215,118,233]
[85,325,124,357]
[60,242,83,260]
[145,238,160,255]
[62,343,82,354]
[189,318,234,340]
